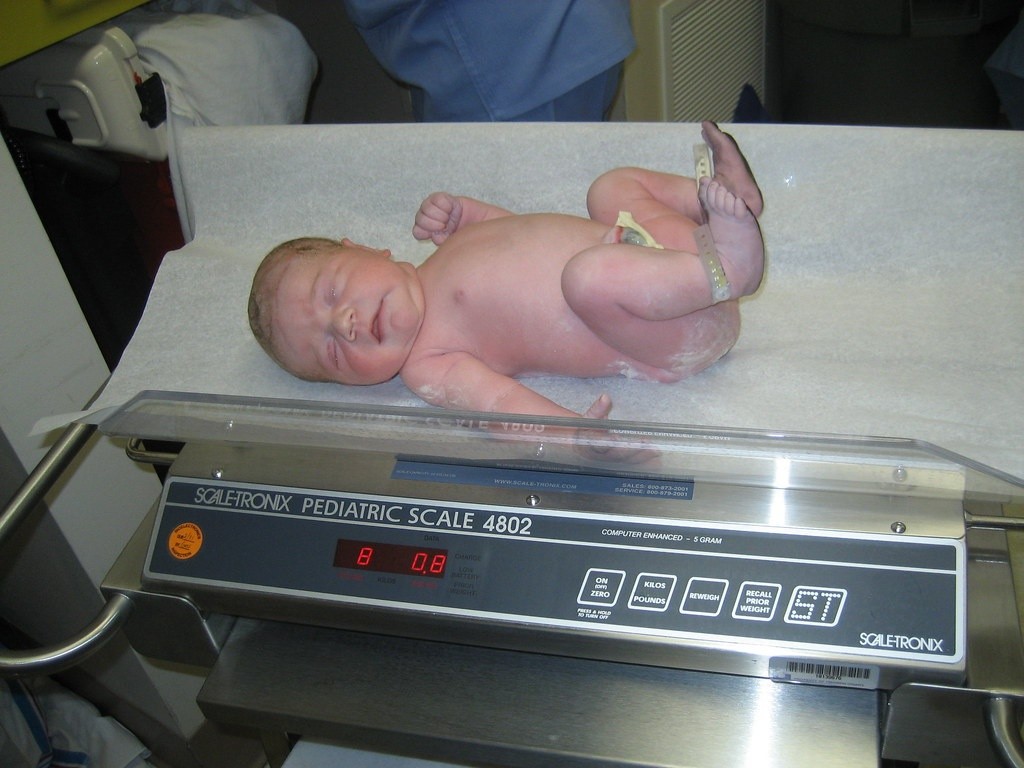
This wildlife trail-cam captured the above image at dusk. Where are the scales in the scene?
[94,161,1024,694]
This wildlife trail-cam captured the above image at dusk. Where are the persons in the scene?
[243,120,767,476]
[338,0,638,127]
